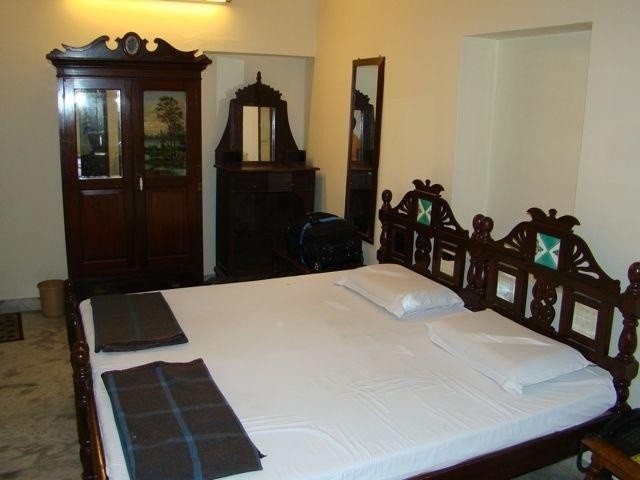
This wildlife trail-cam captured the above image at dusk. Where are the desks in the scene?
[272,245,318,277]
[578,429,640,479]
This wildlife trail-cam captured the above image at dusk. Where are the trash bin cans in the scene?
[37,279,66,317]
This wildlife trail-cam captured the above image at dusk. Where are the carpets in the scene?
[0,311,25,344]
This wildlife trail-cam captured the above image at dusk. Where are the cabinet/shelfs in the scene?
[347,90,376,219]
[44,30,211,297]
[217,67,320,280]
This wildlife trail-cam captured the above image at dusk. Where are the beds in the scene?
[63,177,640,478]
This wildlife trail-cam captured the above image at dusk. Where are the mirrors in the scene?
[343,55,385,245]
[351,109,365,165]
[242,105,278,165]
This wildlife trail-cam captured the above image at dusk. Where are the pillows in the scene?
[333,261,464,319]
[427,306,598,394]
